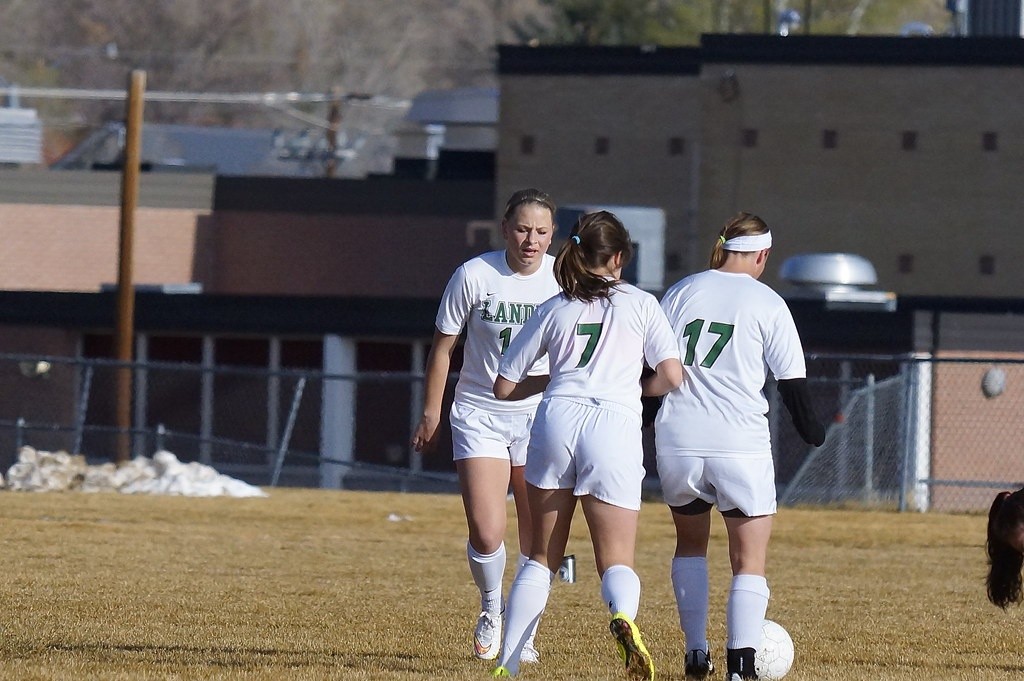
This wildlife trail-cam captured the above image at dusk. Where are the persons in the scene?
[984,485,1024,615]
[653,211,828,681]
[493,210,682,673]
[408,189,565,664]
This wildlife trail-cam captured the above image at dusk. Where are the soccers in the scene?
[727,618,795,681]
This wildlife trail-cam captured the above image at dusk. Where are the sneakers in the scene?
[491,666,517,680]
[515,645,540,663]
[609,614,654,681]
[473,603,506,660]
[678,648,715,680]
[726,671,757,681]
